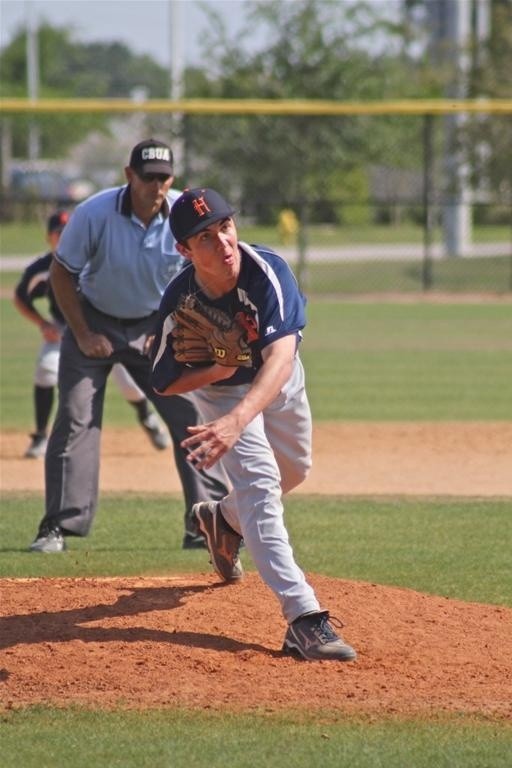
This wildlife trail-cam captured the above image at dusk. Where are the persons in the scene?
[152,185,356,661]
[27,139,246,552]
[13,212,170,458]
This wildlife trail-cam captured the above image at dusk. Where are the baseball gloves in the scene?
[174,295,253,367]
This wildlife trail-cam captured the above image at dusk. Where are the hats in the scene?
[47,210,70,232]
[129,137,175,177]
[168,187,236,245]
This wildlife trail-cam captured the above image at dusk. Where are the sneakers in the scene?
[281,608,357,661]
[140,412,169,451]
[190,499,245,582]
[24,431,49,458]
[29,522,65,553]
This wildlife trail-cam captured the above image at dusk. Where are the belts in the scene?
[81,294,161,328]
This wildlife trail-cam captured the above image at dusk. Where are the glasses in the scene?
[135,170,171,184]
